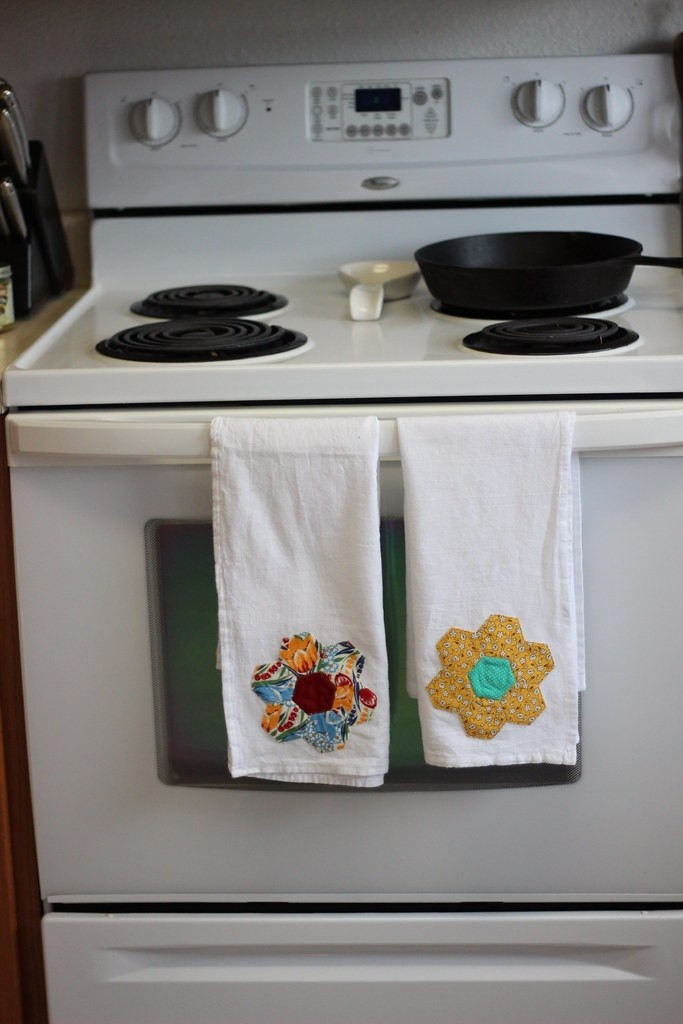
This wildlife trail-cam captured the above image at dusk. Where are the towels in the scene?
[205,410,384,794]
[391,409,594,774]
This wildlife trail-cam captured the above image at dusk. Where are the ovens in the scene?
[2,408,682,911]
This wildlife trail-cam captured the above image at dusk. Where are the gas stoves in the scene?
[16,52,683,364]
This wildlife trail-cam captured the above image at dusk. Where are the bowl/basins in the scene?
[340,260,422,322]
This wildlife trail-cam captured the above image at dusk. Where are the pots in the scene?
[415,231,683,312]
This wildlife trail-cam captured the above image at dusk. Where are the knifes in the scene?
[0,77,32,236]
